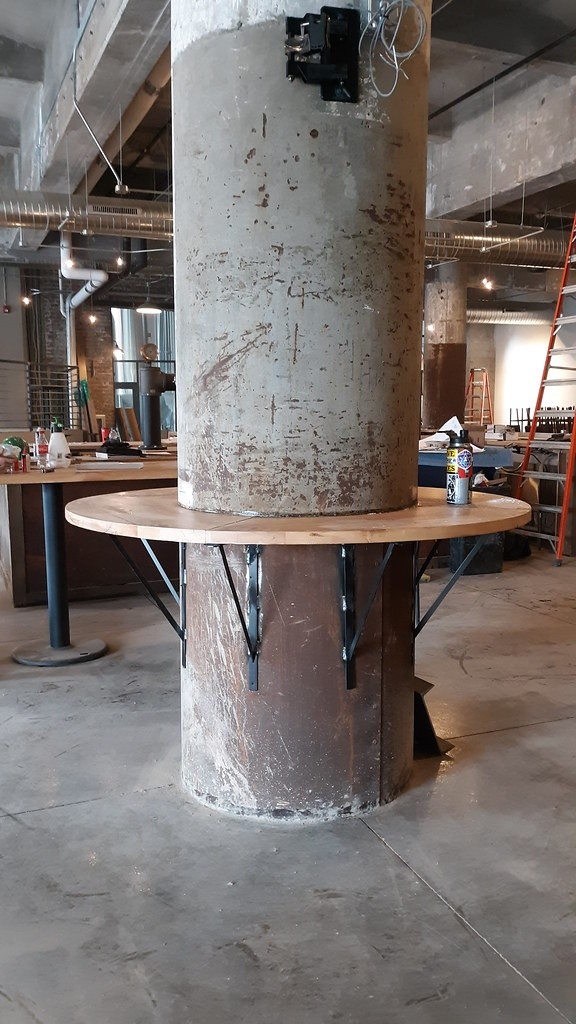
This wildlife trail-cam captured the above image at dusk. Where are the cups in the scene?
[101,428,110,443]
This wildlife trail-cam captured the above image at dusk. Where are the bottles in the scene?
[108,427,117,440]
[37,430,48,468]
[21,448,31,472]
[445,429,473,506]
[47,425,71,468]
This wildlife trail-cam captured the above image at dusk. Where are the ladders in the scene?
[514,213,575,568]
[465,368,495,426]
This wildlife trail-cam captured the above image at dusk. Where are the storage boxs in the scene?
[450,532,504,576]
